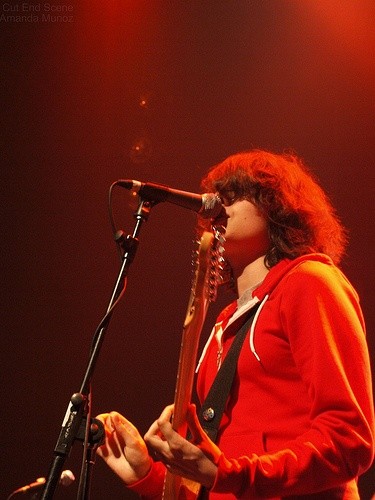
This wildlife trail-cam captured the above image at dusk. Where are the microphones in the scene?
[118,179,222,219]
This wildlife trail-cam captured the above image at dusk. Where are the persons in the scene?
[87,144,375,500]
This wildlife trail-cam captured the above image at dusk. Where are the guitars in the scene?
[155,203,228,500]
